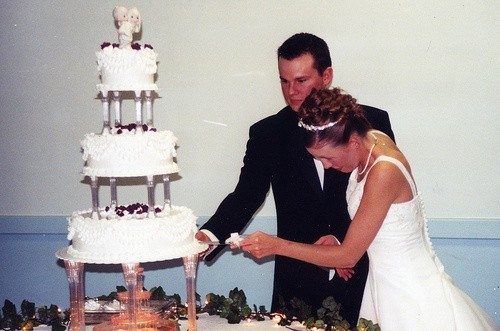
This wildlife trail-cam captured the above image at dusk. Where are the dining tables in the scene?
[0,308,336,331]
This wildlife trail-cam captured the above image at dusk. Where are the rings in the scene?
[248,251,252,255]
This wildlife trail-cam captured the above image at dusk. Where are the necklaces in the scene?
[357,132,377,175]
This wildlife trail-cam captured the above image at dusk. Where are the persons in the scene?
[113,5,142,47]
[229,87,499,331]
[196,32,397,330]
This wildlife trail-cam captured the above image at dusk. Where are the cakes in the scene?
[67,42,202,258]
